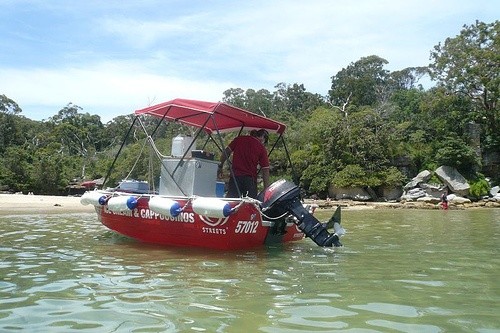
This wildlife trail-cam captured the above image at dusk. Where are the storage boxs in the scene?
[216,181,225,198]
[171,136,197,160]
[191,150,215,161]
[118,181,149,194]
[159,157,222,197]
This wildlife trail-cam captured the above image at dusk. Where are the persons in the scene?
[216,128,271,201]
[440,192,449,208]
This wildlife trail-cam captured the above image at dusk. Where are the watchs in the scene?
[218,164,223,168]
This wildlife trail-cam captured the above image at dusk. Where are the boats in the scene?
[81,98,343,247]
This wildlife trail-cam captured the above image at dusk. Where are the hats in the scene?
[258,128,268,146]
